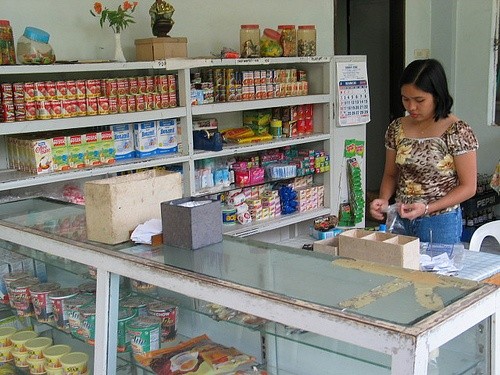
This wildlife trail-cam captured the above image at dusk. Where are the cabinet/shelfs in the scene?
[0,196,500,375]
[0,55,367,246]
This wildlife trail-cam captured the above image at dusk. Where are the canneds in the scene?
[0,75,178,123]
[190,68,314,139]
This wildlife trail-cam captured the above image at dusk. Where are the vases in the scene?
[114,33,127,62]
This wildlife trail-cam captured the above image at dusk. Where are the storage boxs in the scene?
[309,224,421,268]
[231,146,330,222]
[134,36,188,60]
[192,110,272,151]
[83,168,182,244]
[160,196,225,252]
[5,119,179,176]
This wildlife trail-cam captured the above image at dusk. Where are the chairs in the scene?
[465,219,500,255]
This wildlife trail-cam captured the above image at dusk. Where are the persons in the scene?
[371,58,478,252]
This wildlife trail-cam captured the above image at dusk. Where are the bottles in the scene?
[277,25,297,57]
[269,120,282,140]
[194,83,204,105]
[461,172,495,226]
[296,25,316,57]
[259,28,284,57]
[240,24,260,58]
[378,224,387,233]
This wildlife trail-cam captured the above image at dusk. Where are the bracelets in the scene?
[424,202,428,216]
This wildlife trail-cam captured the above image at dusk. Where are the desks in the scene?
[280,242,500,285]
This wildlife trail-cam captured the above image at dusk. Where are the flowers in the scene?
[90,1,139,33]
